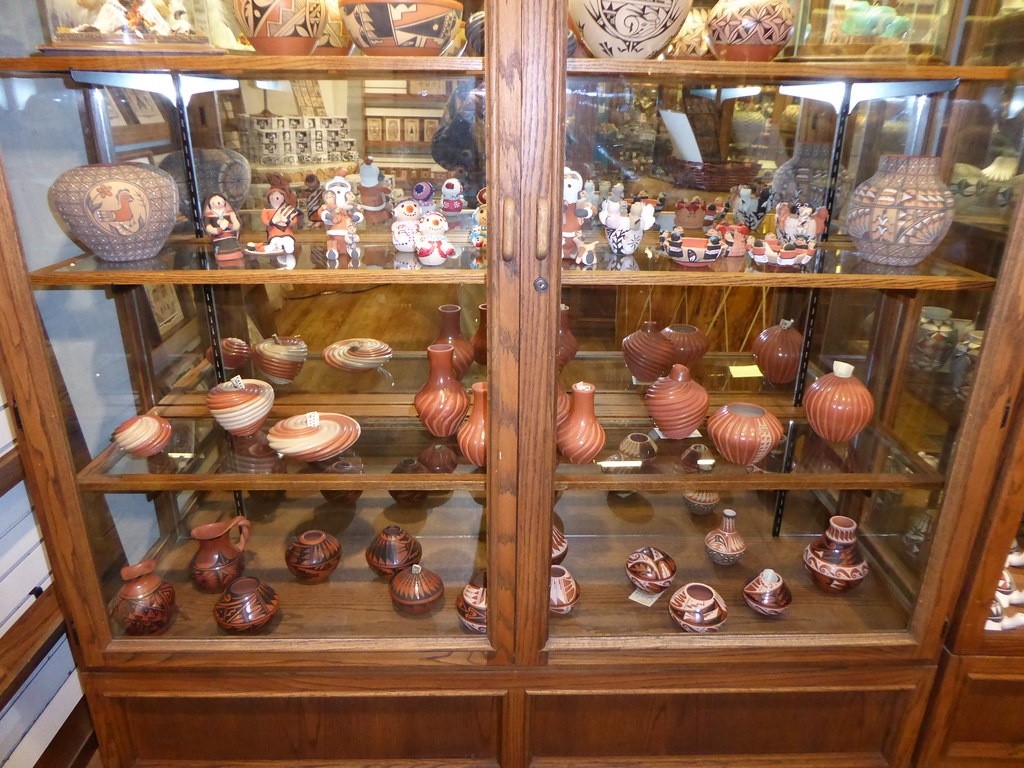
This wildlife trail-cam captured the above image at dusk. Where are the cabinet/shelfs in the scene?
[83,84,210,415]
[0,0,1024,768]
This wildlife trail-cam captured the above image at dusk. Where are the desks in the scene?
[846,340,964,509]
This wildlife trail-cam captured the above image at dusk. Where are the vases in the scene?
[285,529,342,584]
[705,508,747,566]
[114,559,176,637]
[549,565,580,614]
[454,569,487,631]
[804,360,875,443]
[660,323,709,371]
[706,401,783,465]
[391,563,444,614]
[684,460,721,514]
[669,581,727,632]
[915,306,957,368]
[617,433,658,462]
[231,444,455,505]
[803,515,868,592]
[751,317,802,383]
[560,305,576,372]
[364,526,422,578]
[744,565,792,618]
[459,382,487,469]
[847,151,956,268]
[47,161,179,263]
[602,454,639,497]
[471,305,488,365]
[251,334,308,386]
[213,577,279,630]
[551,519,567,560]
[207,376,274,437]
[56,0,797,58]
[436,304,473,380]
[186,514,251,595]
[643,363,709,440]
[555,383,605,463]
[620,321,675,381]
[415,344,468,438]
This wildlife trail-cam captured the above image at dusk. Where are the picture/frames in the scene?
[104,86,133,125]
[138,283,191,343]
[119,87,167,124]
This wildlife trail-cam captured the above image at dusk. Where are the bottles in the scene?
[706,402,783,465]
[553,381,606,464]
[284,530,342,583]
[112,560,177,638]
[742,570,793,616]
[188,515,250,594]
[668,582,729,633]
[703,508,746,566]
[549,565,582,617]
[549,304,806,387]
[802,516,869,594]
[50,161,181,262]
[212,576,281,634]
[906,305,984,402]
[365,524,422,582]
[412,342,488,467]
[161,147,255,231]
[426,303,487,383]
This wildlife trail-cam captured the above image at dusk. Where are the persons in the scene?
[428,74,488,207]
